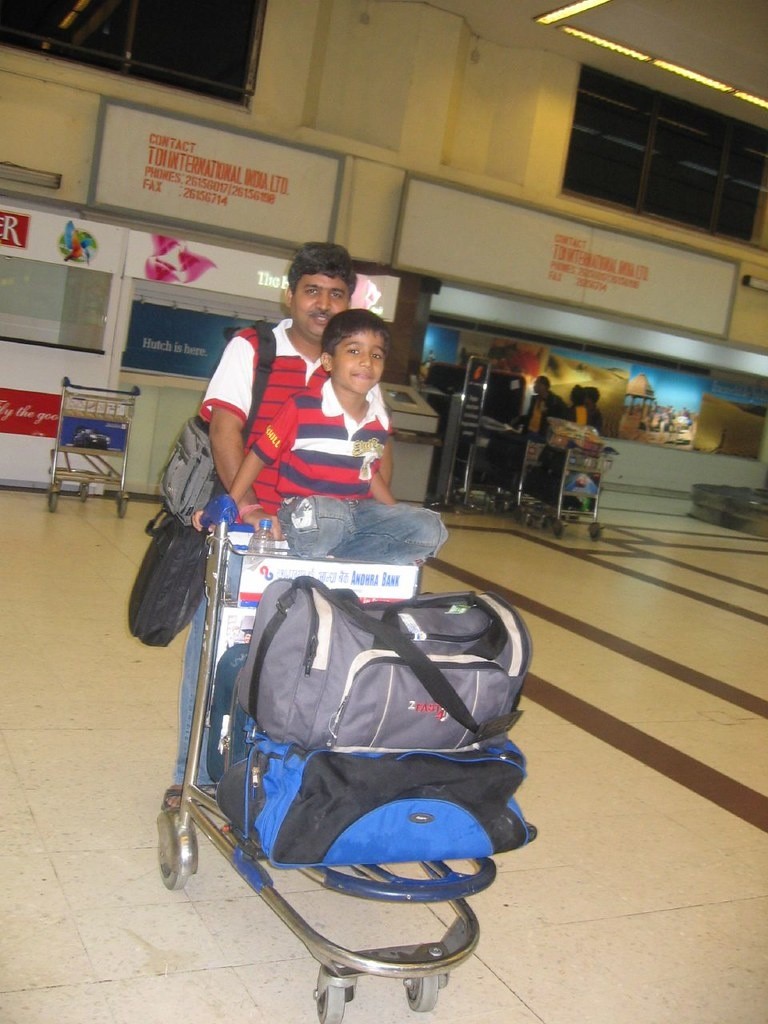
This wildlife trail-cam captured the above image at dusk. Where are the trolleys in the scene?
[49,376,141,518]
[157,493,538,1024]
[514,421,619,542]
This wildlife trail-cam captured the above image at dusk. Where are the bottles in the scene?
[248,519,274,556]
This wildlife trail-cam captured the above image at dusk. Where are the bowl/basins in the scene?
[89,434,110,449]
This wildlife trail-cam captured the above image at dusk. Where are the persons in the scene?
[487,376,525,486]
[161,242,392,808]
[570,385,604,437]
[192,310,448,564]
[521,375,569,441]
[645,398,692,433]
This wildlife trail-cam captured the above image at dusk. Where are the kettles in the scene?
[72,426,97,448]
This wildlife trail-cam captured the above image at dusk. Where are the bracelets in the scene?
[239,504,262,519]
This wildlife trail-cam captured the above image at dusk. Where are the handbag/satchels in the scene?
[238,576,533,752]
[217,703,538,868]
[162,416,220,526]
[127,506,208,646]
